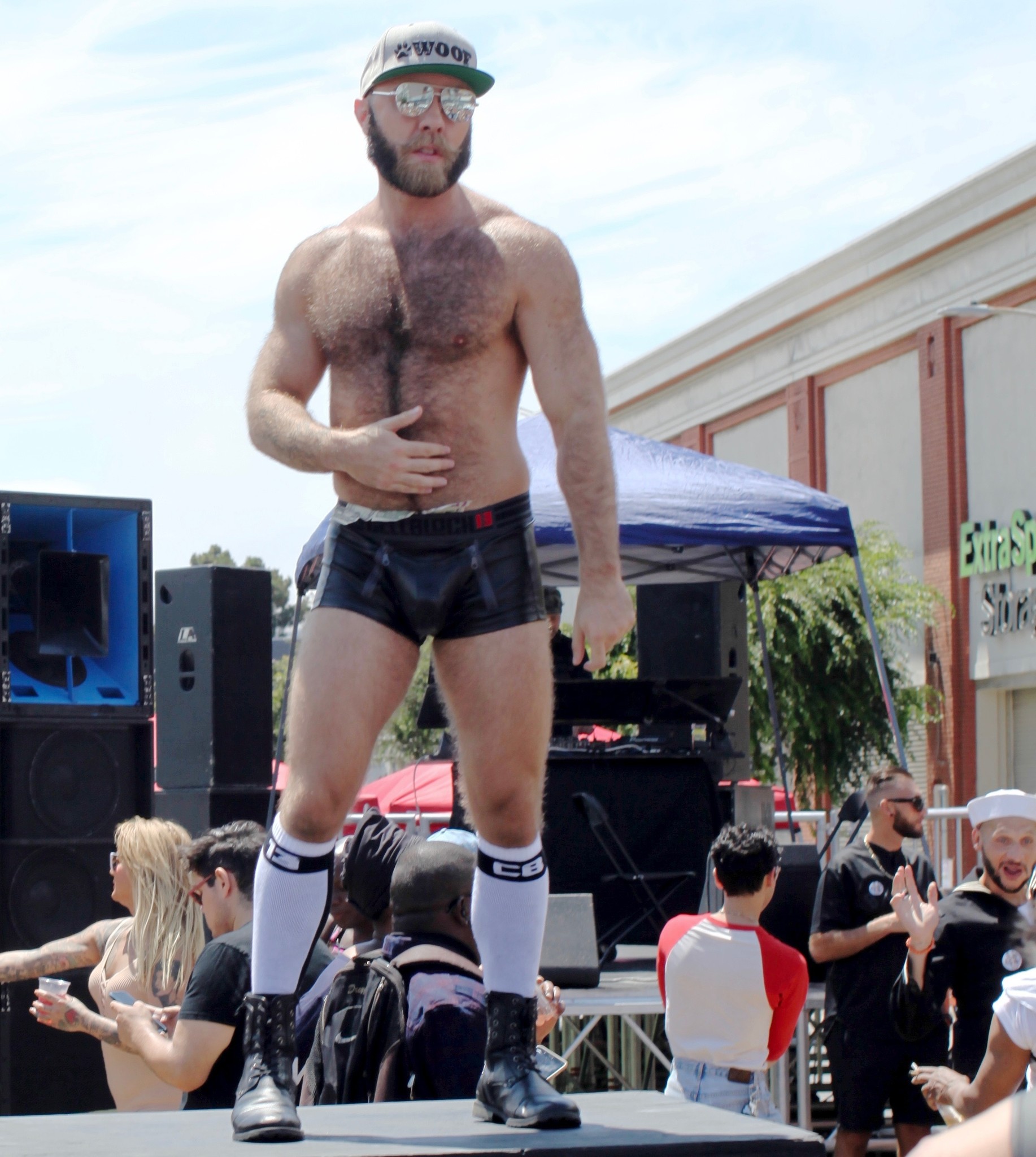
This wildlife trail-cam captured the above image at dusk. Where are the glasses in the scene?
[188,873,213,905]
[371,81,479,124]
[110,851,120,872]
[887,795,924,812]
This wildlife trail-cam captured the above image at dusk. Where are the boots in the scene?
[471,990,583,1130]
[231,992,304,1143]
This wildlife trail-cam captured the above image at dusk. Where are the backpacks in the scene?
[298,942,485,1107]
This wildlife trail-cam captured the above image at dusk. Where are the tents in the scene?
[269,413,936,926]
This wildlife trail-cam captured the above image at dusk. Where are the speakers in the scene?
[0,491,279,1012]
[636,578,829,982]
[539,893,600,990]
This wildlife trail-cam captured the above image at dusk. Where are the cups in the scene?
[37,977,71,1025]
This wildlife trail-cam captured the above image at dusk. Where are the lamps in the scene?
[935,303,1036,321]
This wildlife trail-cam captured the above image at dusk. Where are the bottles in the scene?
[479,963,558,1026]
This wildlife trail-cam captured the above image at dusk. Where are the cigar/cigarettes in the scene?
[908,1070,919,1076]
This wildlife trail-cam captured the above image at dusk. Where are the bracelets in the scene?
[906,935,935,953]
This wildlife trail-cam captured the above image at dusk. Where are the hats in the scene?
[542,585,563,613]
[359,22,494,98]
[966,787,1036,827]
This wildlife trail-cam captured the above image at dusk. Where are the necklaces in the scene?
[863,834,909,880]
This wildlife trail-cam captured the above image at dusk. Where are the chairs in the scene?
[815,793,869,865]
[566,788,695,970]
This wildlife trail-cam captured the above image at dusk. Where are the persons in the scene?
[656,822,810,1124]
[543,585,594,738]
[233,25,636,1139]
[0,814,566,1111]
[809,766,1036,1157]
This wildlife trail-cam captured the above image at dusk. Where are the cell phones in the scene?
[109,991,167,1033]
[534,1044,566,1083]
